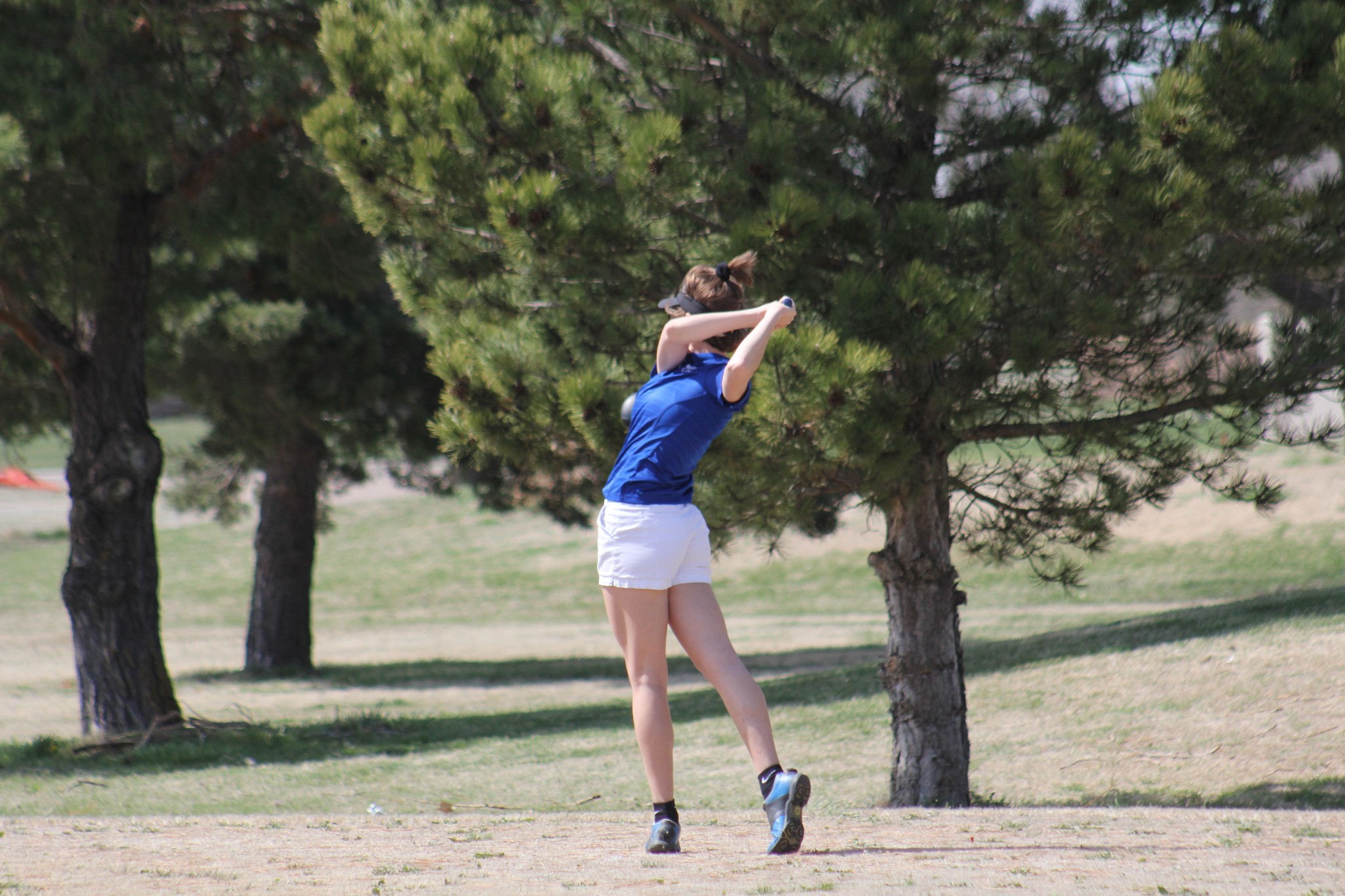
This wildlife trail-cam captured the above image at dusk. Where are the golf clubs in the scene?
[620,298,794,427]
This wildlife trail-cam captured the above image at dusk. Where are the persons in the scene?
[597,249,812,856]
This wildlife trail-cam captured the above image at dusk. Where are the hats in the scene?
[657,292,730,335]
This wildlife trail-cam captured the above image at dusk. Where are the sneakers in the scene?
[645,817,682,854]
[763,769,810,855]
